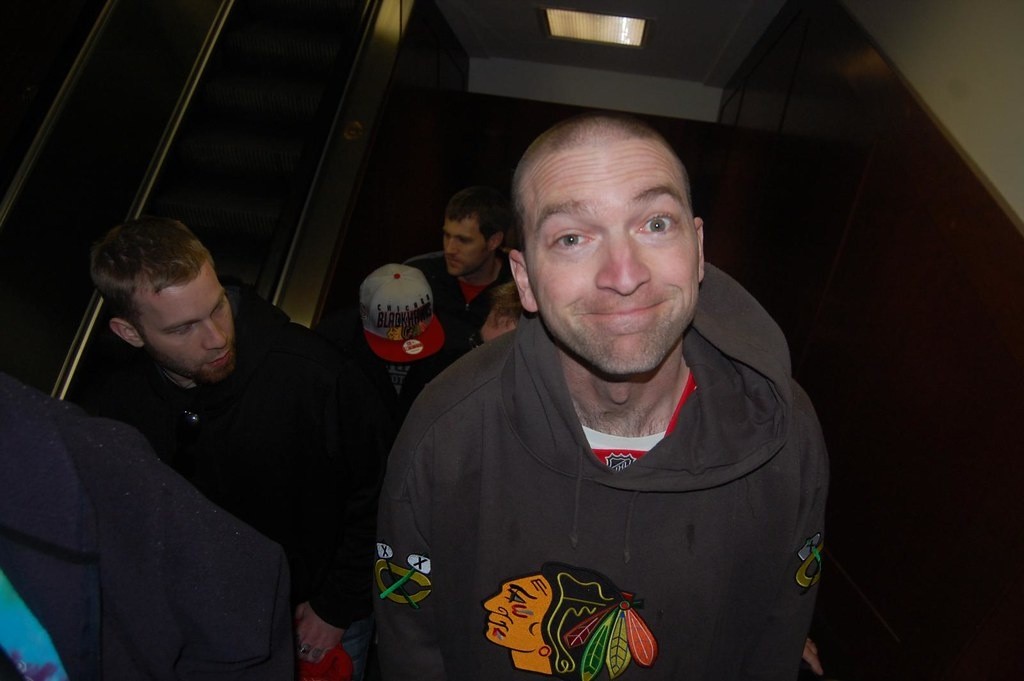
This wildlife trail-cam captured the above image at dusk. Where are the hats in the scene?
[359,264,445,362]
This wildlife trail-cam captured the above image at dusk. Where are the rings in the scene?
[310,648,322,659]
[299,644,310,655]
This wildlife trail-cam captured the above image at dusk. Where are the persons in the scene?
[0,186,822,681]
[375,116,827,681]
